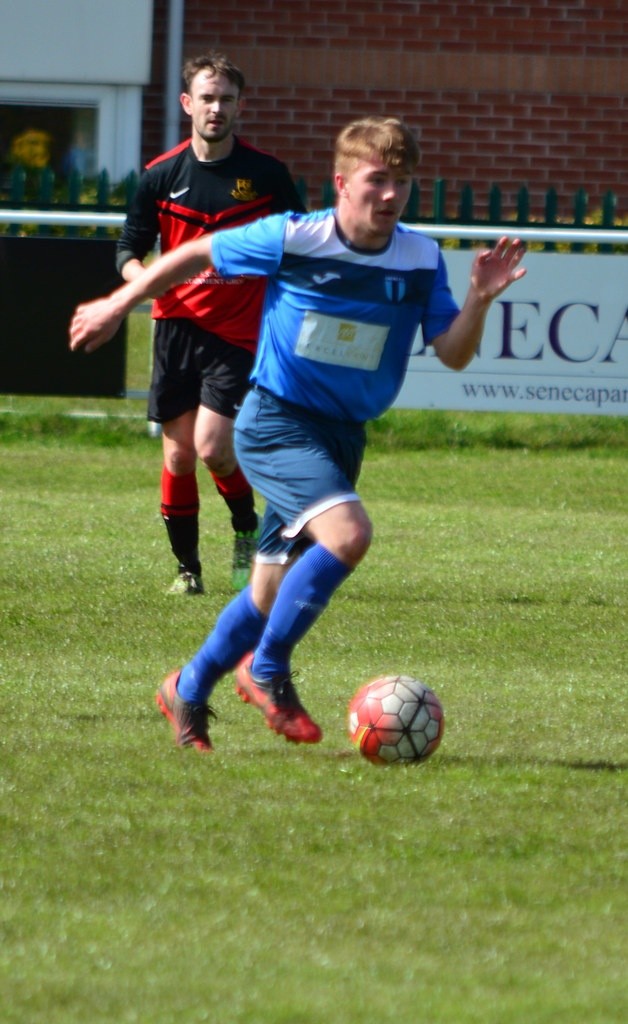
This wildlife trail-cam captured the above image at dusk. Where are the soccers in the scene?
[344,674,446,768]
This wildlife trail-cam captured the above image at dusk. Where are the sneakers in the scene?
[155,670,216,757]
[165,571,204,598]
[232,650,323,745]
[230,513,264,591]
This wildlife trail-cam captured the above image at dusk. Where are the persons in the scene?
[69,118,527,749]
[116,54,309,592]
